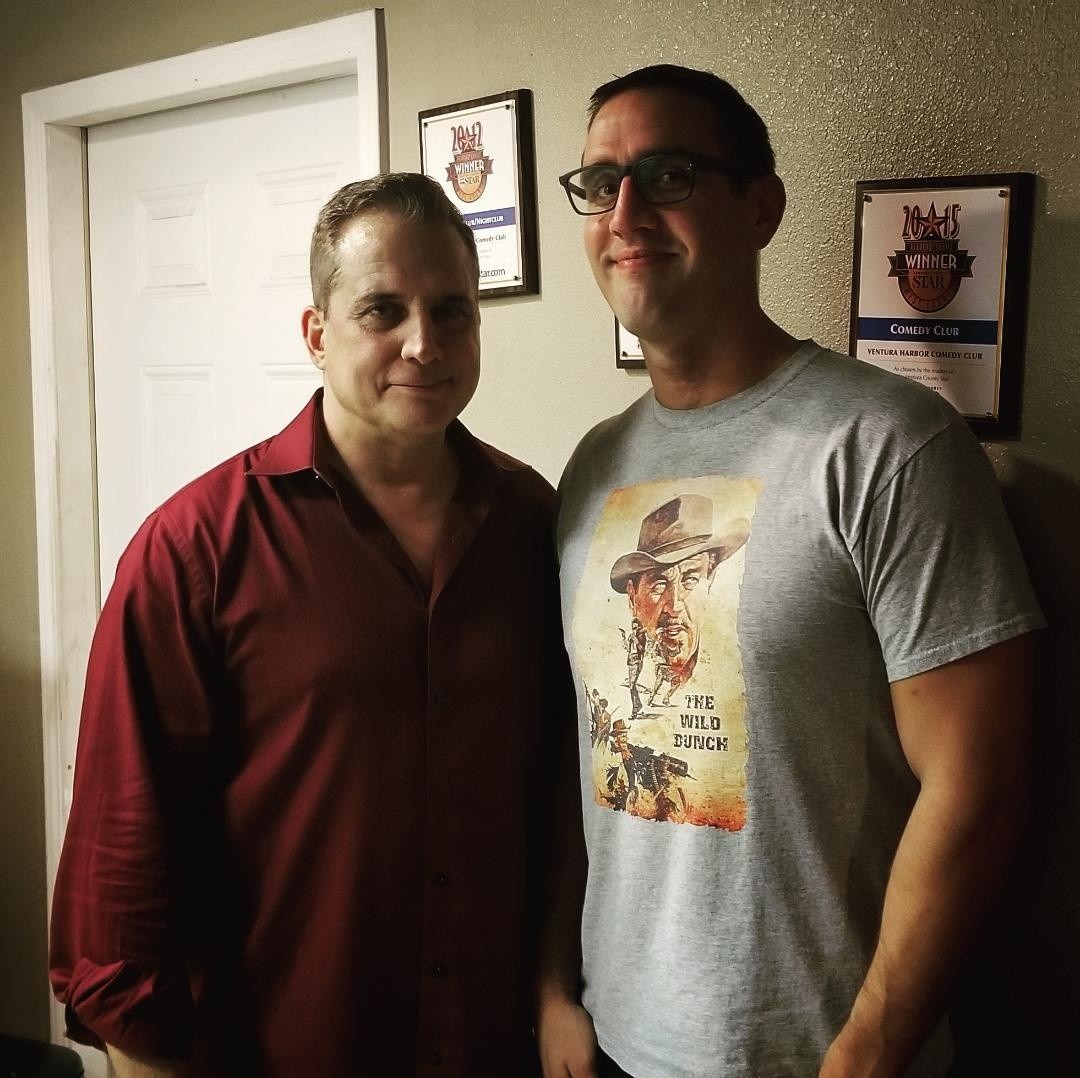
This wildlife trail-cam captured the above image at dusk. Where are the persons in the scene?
[50,173,555,1078]
[536,64,1056,1078]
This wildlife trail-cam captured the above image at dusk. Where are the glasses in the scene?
[559,150,775,216]
